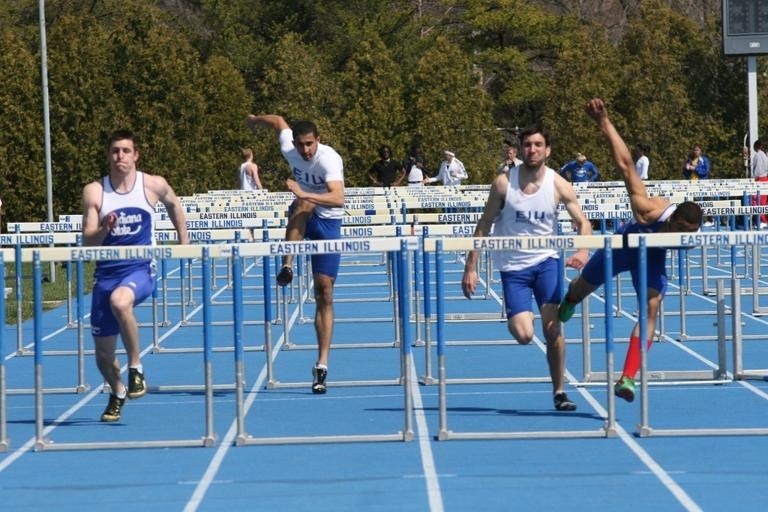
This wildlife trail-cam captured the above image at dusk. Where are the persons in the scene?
[239,147,263,190]
[635,139,650,181]
[495,145,523,175]
[403,144,434,187]
[560,97,704,402]
[247,113,345,394]
[750,139,768,229]
[422,150,469,186]
[560,151,600,183]
[366,145,406,186]
[81,128,191,421]
[683,143,715,227]
[462,124,593,411]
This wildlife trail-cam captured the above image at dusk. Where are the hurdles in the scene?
[20,243,221,451]
[628,230,768,437]
[567,276,733,386]
[7,220,133,356]
[558,178,768,342]
[252,225,411,393]
[103,228,252,393]
[56,214,182,327]
[220,236,419,448]
[730,275,768,381]
[411,221,574,386]
[153,182,544,354]
[0,232,92,395]
[0,247,15,450]
[423,234,623,442]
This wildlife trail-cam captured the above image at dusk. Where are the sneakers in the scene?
[553,391,576,410]
[276,266,294,287]
[614,375,635,403]
[311,363,328,394]
[558,294,576,323]
[101,391,126,421]
[127,366,146,399]
